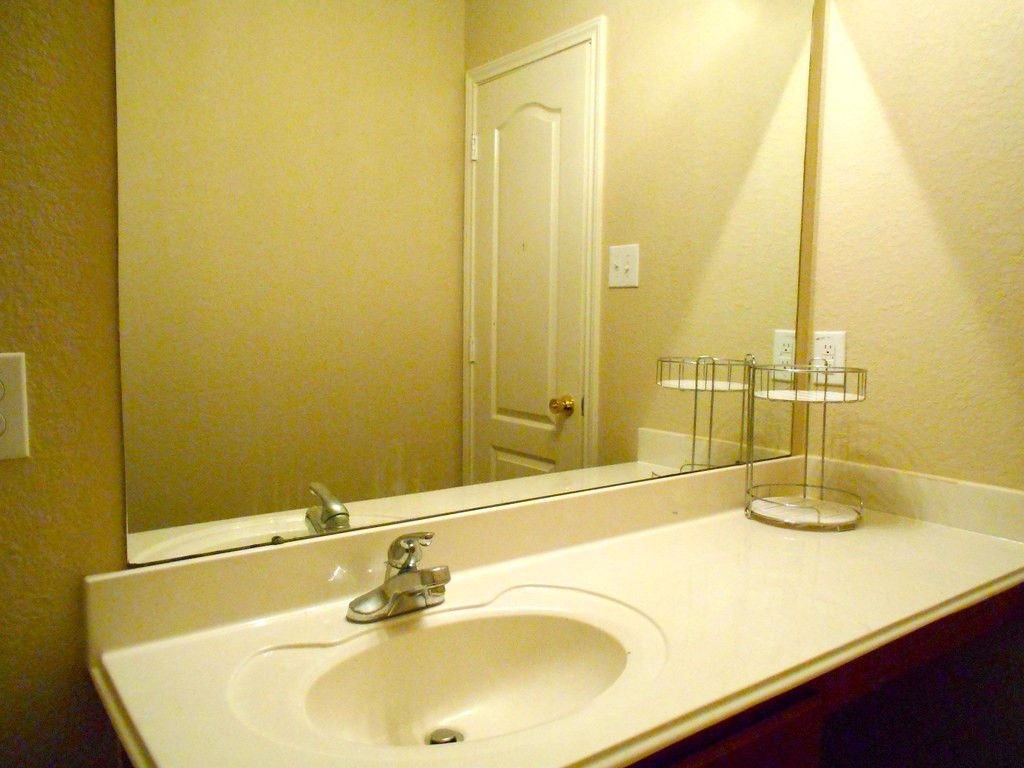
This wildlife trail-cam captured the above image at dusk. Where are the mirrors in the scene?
[109,1,815,566]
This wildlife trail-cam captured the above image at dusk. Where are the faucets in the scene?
[347,531,452,624]
[304,478,350,548]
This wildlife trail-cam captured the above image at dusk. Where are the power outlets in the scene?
[771,329,795,383]
[812,331,846,387]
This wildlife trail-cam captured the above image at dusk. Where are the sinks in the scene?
[300,610,630,768]
